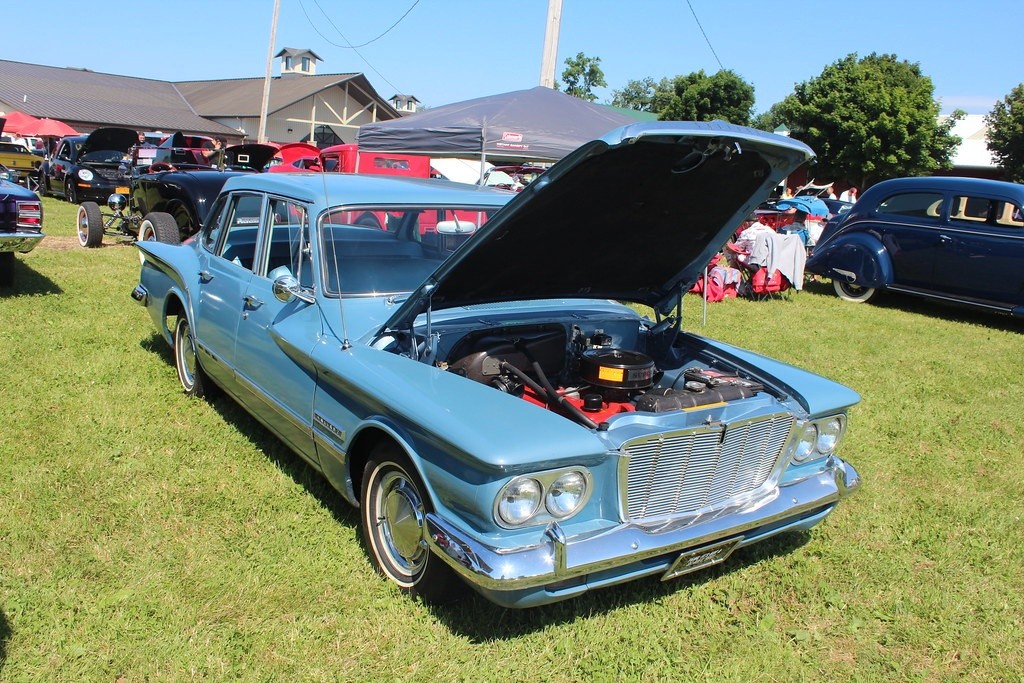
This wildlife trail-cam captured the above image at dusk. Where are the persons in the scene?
[201,135,228,164]
[133,130,152,147]
[780,184,858,214]
[730,211,776,271]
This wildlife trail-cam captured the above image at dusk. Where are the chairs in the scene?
[726,228,816,300]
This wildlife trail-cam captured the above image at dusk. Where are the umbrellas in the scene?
[0,111,40,133]
[16,117,80,175]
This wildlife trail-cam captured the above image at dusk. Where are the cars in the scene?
[35,126,857,269]
[131,118,864,611]
[805,174,1024,322]
[0,163,46,253]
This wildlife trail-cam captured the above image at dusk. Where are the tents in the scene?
[347,86,639,230]
